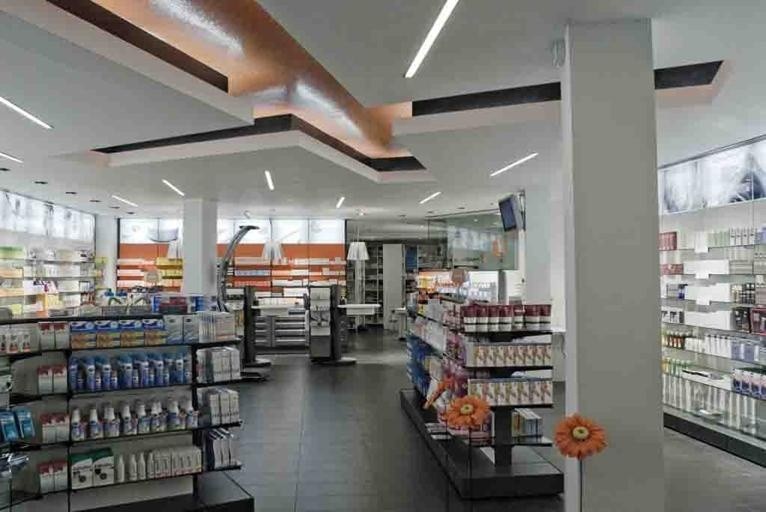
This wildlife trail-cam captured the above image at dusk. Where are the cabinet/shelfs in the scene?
[0,239,186,317]
[226,264,348,356]
[398,292,565,512]
[657,195,766,471]
[0,312,255,511]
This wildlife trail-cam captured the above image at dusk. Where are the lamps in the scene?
[346,208,371,261]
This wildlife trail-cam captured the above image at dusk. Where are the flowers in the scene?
[447,395,488,510]
[556,415,605,512]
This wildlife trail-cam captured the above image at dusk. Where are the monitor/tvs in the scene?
[497,195,522,233]
